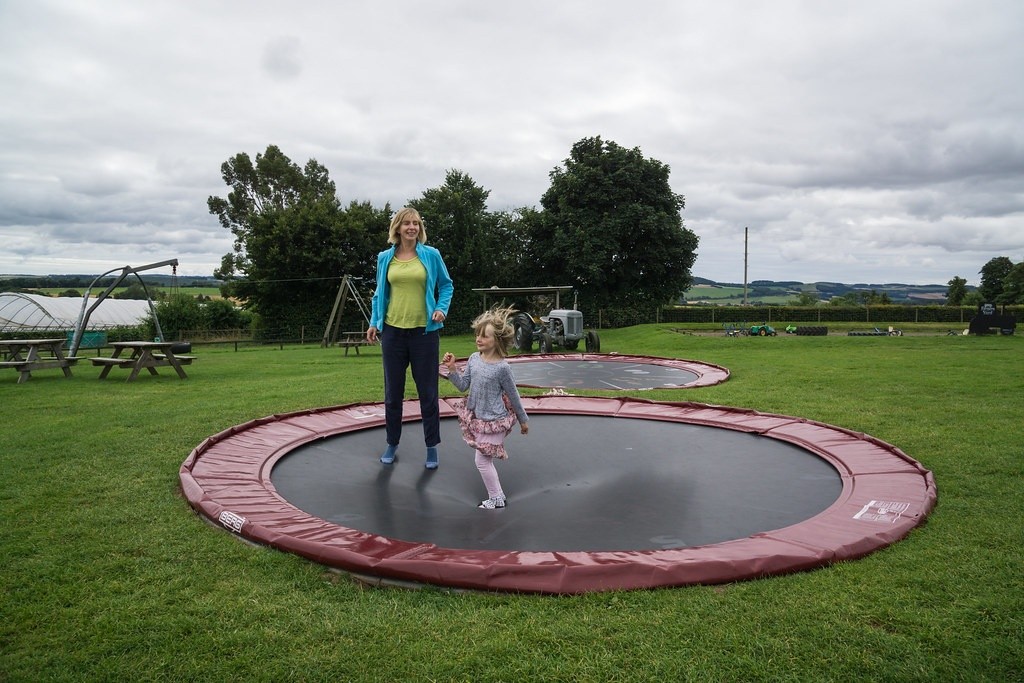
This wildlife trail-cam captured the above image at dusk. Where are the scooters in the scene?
[722,320,750,337]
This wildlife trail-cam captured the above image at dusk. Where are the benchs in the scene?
[337,341,382,344]
[22,356,86,360]
[0,362,33,366]
[86,357,135,364]
[151,353,197,361]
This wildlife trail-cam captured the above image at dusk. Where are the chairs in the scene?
[539,316,550,325]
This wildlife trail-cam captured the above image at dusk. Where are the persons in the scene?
[441,301,529,508]
[365,208,454,468]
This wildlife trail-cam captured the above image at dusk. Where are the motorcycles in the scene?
[748,321,778,336]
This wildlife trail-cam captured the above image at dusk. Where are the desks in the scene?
[343,331,383,357]
[0,338,72,383]
[93,341,191,383]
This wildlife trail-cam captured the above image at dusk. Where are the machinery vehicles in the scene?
[513,308,601,355]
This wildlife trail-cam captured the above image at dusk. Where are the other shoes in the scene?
[478,494,506,510]
[380,441,399,463]
[425,446,439,469]
